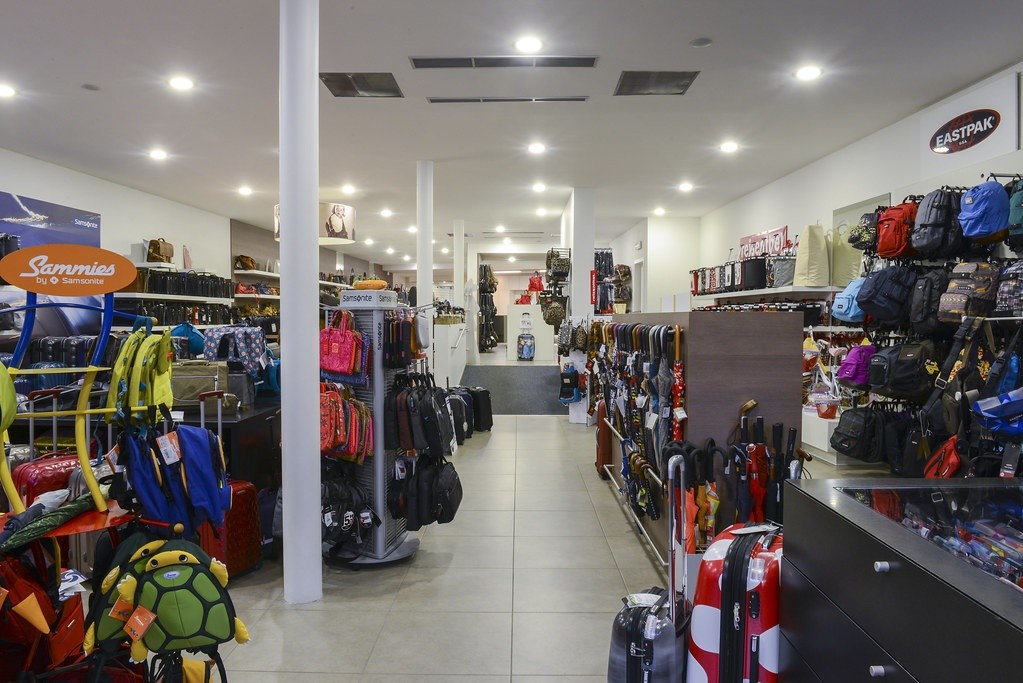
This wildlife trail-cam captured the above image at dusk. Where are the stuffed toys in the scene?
[83,540,252,664]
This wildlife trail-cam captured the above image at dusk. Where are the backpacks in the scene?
[0,317,248,683]
[793,178,1023,480]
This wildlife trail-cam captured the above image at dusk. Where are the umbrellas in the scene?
[658,397,812,554]
[585,322,688,521]
[0,487,108,555]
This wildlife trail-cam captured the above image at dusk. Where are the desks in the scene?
[11,400,282,494]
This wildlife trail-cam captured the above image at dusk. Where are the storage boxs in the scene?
[229,373,254,405]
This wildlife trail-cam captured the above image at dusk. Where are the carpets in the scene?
[460,366,569,415]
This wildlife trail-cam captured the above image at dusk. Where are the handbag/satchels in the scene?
[538,249,569,326]
[557,320,588,407]
[256,264,498,559]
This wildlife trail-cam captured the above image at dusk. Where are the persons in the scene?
[326,205,348,239]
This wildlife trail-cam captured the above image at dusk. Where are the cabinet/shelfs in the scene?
[101,262,234,358]
[778,476,1023,683]
[693,286,865,331]
[234,269,354,299]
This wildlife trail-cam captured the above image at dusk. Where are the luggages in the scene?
[609,455,686,683]
[517,334,536,361]
[198,390,262,574]
[10,391,82,510]
[687,521,784,683]
